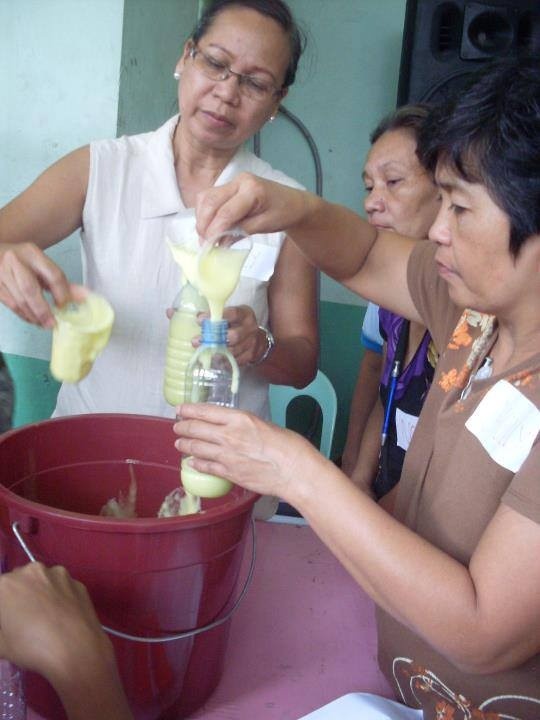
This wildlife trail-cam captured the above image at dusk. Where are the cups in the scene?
[47,290,116,384]
[170,209,253,302]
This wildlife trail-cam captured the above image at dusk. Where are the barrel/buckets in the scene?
[0,411,265,719]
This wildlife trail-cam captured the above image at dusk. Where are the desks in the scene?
[191,518,394,720]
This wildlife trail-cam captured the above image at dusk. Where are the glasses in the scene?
[189,46,282,103]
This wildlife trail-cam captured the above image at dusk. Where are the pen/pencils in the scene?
[461,356,492,402]
[381,359,400,446]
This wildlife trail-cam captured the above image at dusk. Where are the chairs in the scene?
[264,366,337,524]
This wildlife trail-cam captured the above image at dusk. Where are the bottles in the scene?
[180,317,242,498]
[161,278,211,408]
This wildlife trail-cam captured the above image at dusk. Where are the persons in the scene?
[172,51,540,720]
[0,0,322,524]
[0,564,131,720]
[338,105,443,504]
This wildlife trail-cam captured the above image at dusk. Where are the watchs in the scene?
[245,325,275,368]
